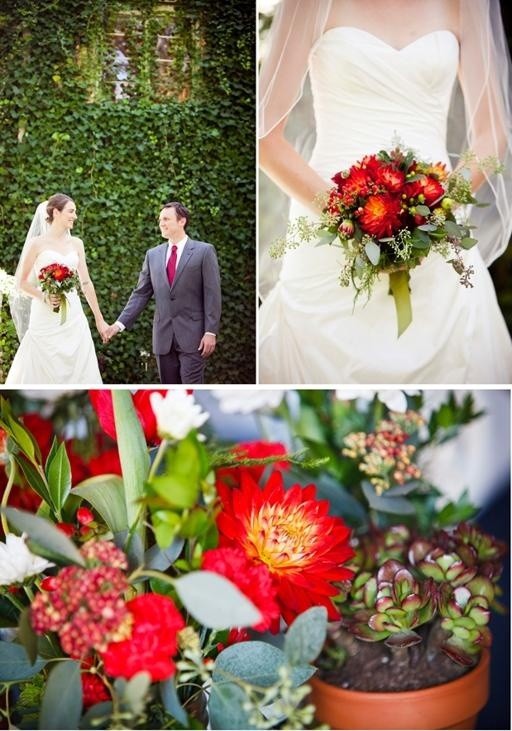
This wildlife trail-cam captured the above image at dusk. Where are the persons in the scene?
[104,200,224,384]
[5,194,114,388]
[255,1,512,390]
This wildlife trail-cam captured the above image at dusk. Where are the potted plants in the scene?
[307,511,504,730]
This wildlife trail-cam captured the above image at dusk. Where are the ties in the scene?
[165,246,178,286]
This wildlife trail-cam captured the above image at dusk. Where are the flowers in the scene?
[0,389,489,685]
[37,263,79,326]
[268,146,505,339]
